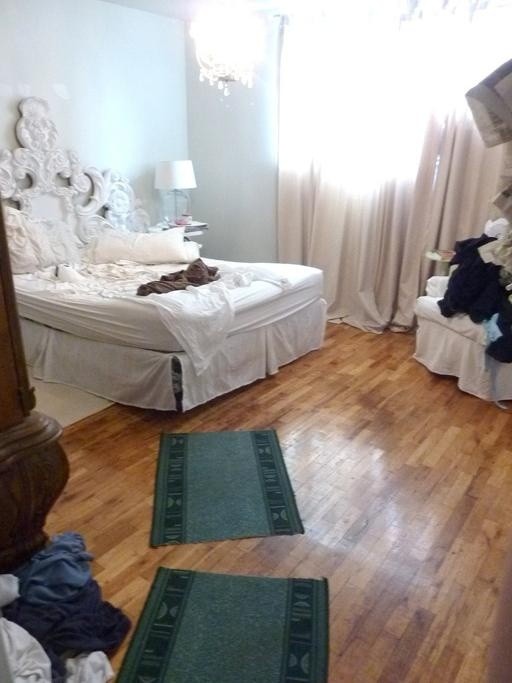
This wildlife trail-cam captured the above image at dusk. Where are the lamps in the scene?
[186,1,276,91]
[153,154,198,232]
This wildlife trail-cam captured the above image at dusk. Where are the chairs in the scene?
[412,275,512,411]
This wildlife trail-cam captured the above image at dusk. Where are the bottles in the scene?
[182,214,192,225]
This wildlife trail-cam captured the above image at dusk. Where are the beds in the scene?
[2,95,329,413]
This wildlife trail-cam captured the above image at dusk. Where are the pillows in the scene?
[92,221,184,263]
[133,238,202,265]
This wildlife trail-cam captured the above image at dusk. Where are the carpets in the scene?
[148,428,306,545]
[114,566,328,683]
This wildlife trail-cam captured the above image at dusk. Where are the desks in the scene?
[157,219,209,243]
[425,248,456,274]
